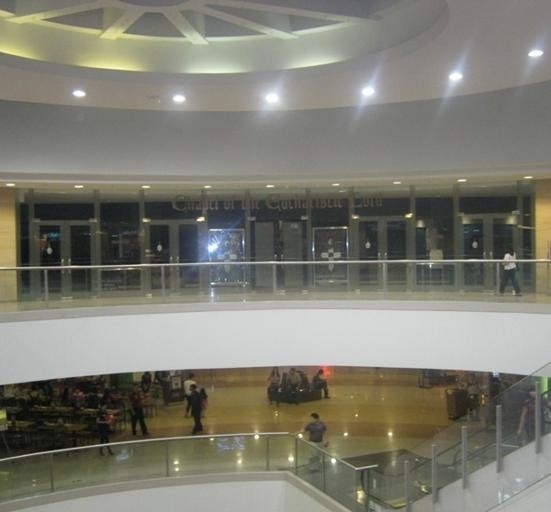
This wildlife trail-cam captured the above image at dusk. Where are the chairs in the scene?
[271,371,322,404]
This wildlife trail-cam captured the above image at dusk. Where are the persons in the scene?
[465,372,511,417]
[294,413,328,470]
[268,367,331,407]
[516,387,551,441]
[184,374,207,433]
[500,245,521,296]
[0,372,171,456]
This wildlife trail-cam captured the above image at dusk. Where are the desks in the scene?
[4,381,162,451]
